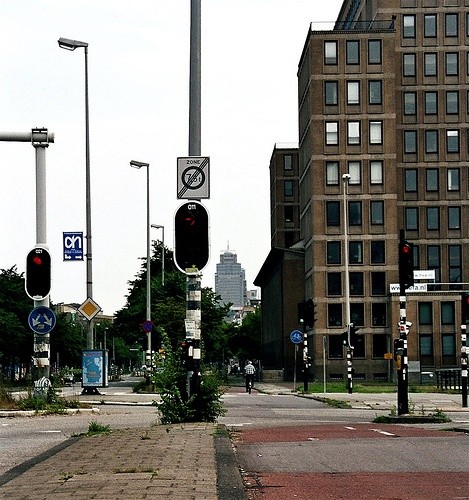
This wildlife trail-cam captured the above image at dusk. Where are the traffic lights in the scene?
[349,322,360,349]
[24,247,51,301]
[298,302,305,324]
[341,331,349,347]
[400,240,414,288]
[461,293,469,325]
[305,299,318,328]
[174,201,209,276]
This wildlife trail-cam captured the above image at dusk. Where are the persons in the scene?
[244,361,255,392]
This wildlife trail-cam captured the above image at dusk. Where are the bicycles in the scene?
[247,377,251,393]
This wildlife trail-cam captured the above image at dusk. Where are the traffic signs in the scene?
[27,306,57,335]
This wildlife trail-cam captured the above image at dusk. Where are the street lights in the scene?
[57,37,101,396]
[130,160,152,385]
[151,224,166,288]
[341,173,352,349]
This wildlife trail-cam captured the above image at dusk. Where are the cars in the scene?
[60,372,75,384]
